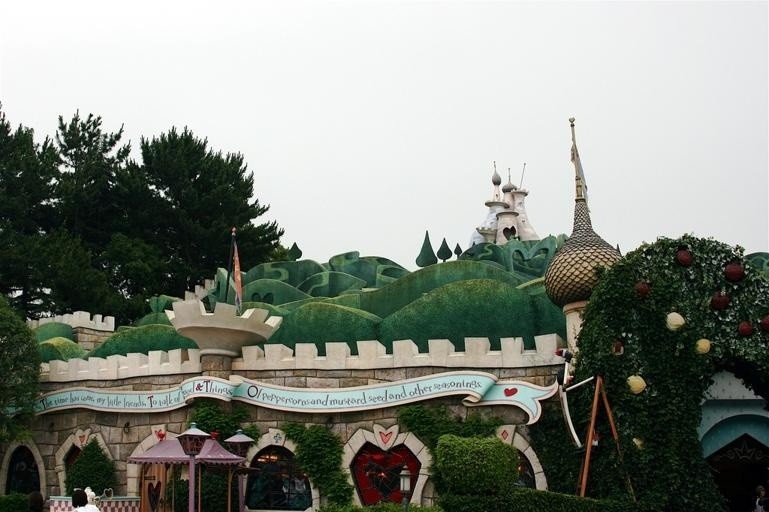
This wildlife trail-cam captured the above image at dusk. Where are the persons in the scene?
[71,489,101,512]
